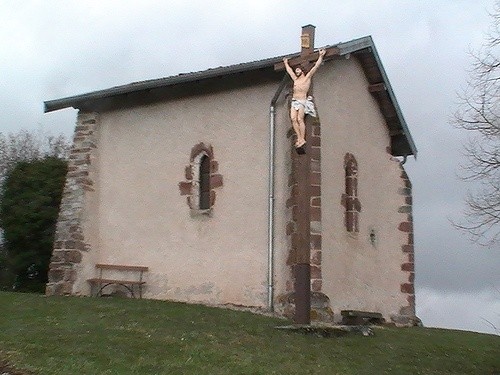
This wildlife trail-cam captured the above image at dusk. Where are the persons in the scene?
[281,48,326,148]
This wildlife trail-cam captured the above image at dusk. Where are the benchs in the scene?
[340,310,383,326]
[87,264,148,300]
